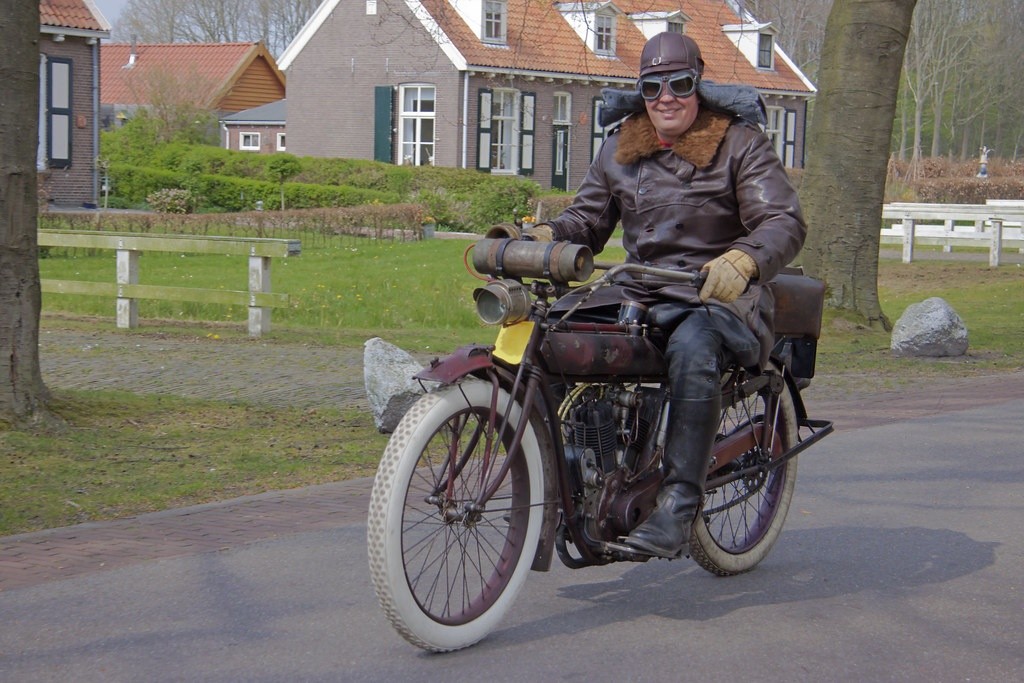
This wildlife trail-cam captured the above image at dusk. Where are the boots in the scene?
[623,393,722,558]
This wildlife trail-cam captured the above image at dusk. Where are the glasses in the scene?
[640,71,696,100]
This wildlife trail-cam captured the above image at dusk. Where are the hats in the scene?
[637,32,704,81]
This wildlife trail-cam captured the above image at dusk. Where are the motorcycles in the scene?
[362,224,835,654]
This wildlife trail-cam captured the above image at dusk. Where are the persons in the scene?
[531,31,808,559]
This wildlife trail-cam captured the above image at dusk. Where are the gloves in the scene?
[520,222,553,243]
[699,249,758,303]
